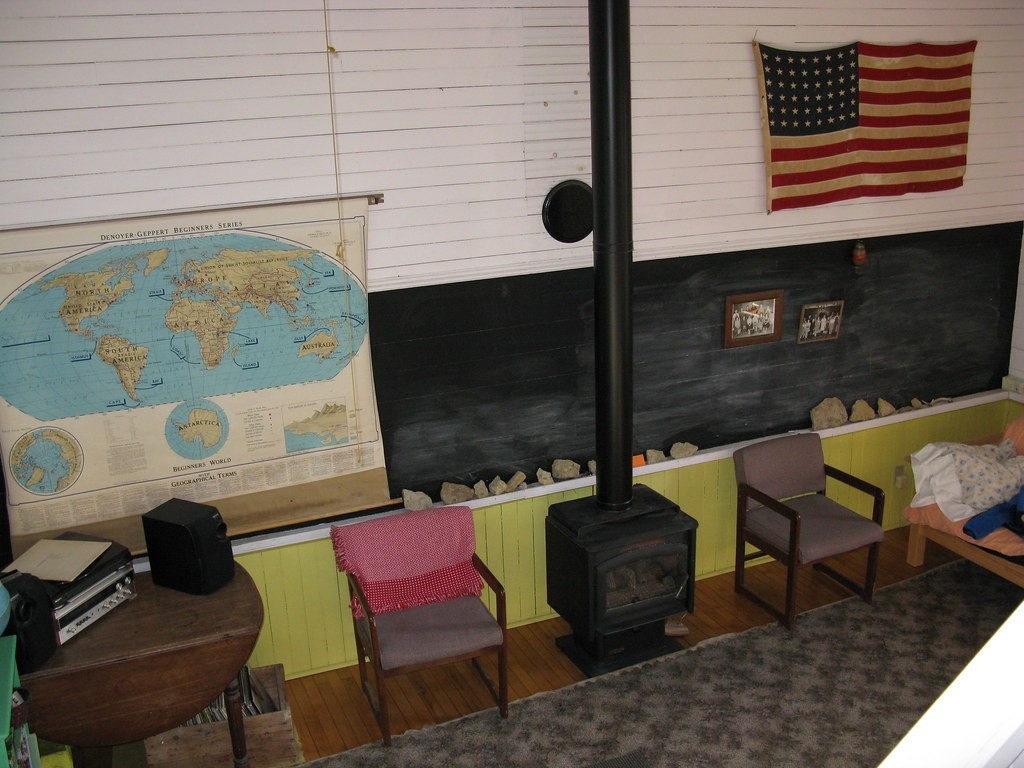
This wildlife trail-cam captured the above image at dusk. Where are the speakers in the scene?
[142,499,236,596]
[0,569,57,675]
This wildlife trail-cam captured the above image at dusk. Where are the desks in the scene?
[0,558,264,768]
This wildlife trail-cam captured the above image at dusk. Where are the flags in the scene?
[753,39,980,215]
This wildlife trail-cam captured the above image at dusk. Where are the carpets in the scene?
[292,559,1024,768]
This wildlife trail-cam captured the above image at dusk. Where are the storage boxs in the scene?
[144,663,295,768]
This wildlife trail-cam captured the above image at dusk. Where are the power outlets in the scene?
[894,464,904,481]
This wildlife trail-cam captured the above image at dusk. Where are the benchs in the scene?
[903,415,1024,589]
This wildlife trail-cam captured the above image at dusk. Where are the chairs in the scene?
[733,433,885,632]
[329,506,509,748]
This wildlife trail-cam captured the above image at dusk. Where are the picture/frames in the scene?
[797,300,844,344]
[724,288,782,350]
